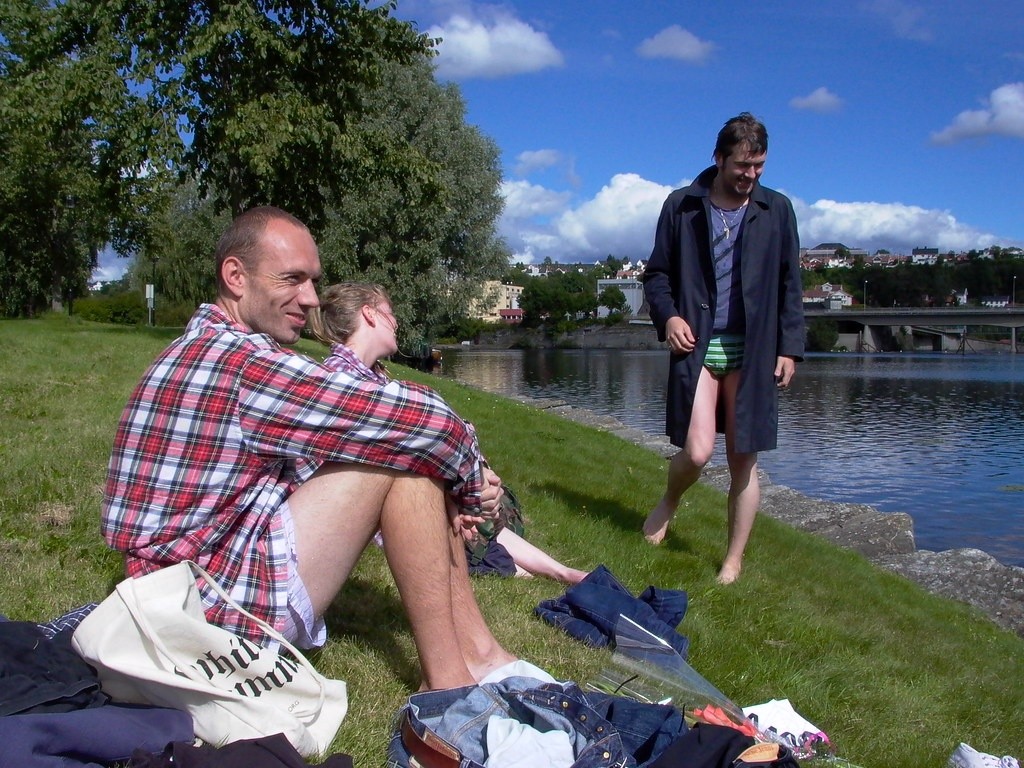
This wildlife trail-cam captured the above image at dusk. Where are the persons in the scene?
[99,206,591,691]
[641,113,806,584]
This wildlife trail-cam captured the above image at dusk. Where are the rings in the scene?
[669,346,672,349]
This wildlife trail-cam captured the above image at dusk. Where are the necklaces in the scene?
[713,184,744,239]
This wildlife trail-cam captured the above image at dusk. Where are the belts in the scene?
[399,698,461,768]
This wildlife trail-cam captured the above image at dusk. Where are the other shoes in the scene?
[951,743,1020,768]
[733,744,799,768]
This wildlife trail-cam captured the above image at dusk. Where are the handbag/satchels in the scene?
[73,563,349,761]
